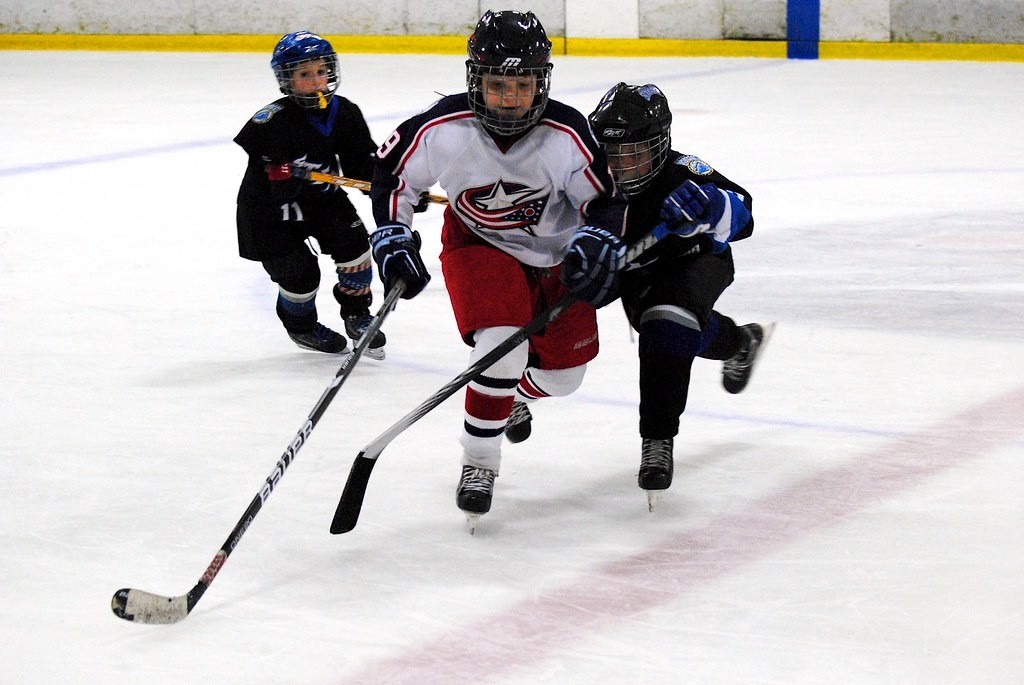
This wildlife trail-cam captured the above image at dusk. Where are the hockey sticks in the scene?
[289,164,450,205]
[110,228,423,628]
[327,181,723,536]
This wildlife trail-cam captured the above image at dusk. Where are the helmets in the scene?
[271,31,341,111]
[588,81,672,201]
[466,9,554,135]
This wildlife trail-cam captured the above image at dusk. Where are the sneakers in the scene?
[340,308,386,360]
[638,439,673,513]
[722,320,777,394]
[455,464,498,535]
[505,401,533,444]
[287,322,351,354]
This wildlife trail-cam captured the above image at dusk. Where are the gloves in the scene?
[660,180,726,237]
[412,191,430,213]
[370,224,431,311]
[559,226,627,309]
[273,177,301,205]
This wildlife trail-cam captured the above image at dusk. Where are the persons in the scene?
[585,81,777,514]
[367,10,619,534]
[234,30,430,360]
